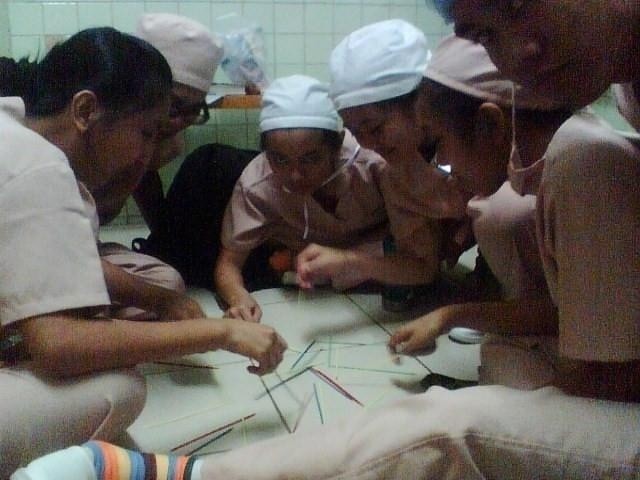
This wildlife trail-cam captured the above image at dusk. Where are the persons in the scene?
[0,26,287,475]
[214,74,387,323]
[75,12,226,321]
[389,37,640,356]
[84,1,639,479]
[294,19,475,291]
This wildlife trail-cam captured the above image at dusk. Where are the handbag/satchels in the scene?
[133,145,276,290]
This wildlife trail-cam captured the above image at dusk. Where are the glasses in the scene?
[169,99,209,125]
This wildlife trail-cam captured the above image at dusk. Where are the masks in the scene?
[506,79,547,198]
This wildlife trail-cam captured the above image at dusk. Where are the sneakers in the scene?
[381,284,413,312]
[449,326,485,345]
[420,374,479,393]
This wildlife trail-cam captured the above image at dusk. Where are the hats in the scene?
[423,30,568,113]
[327,17,433,112]
[259,74,346,136]
[131,12,226,95]
